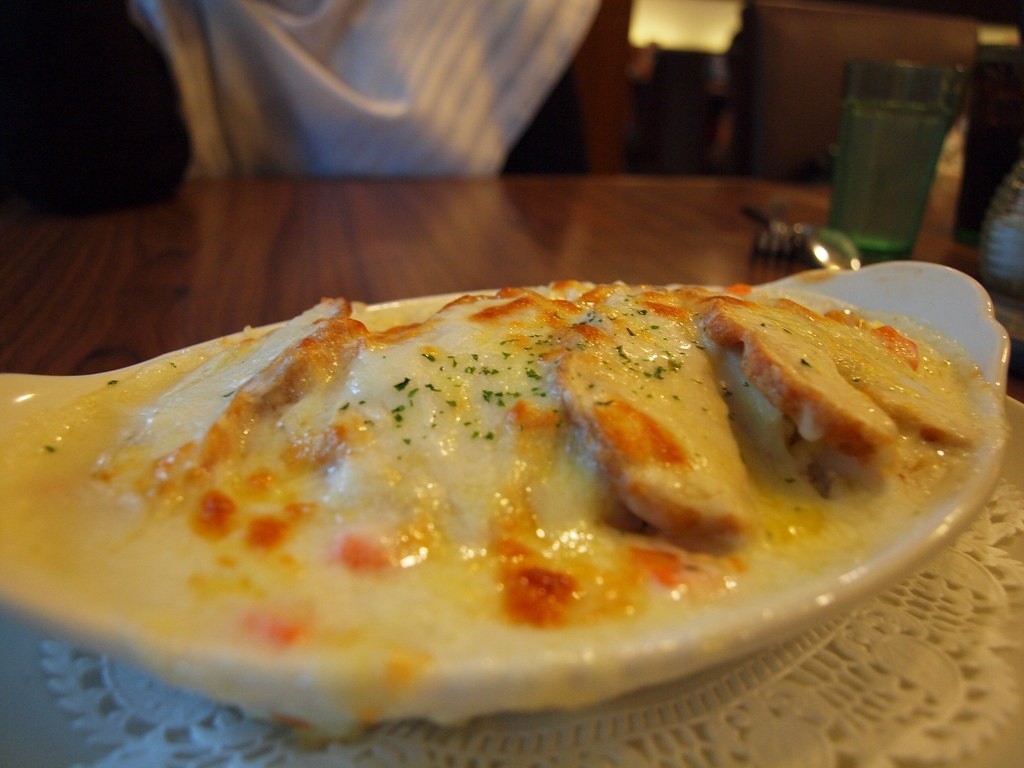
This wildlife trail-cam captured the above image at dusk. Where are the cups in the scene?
[825,46,963,257]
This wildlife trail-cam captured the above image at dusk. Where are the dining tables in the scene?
[0,172,1023,766]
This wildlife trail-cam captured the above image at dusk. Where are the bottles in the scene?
[986,156,1024,299]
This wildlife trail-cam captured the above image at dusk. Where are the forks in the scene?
[729,198,799,253]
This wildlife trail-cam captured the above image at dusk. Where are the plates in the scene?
[2,264,1007,734]
